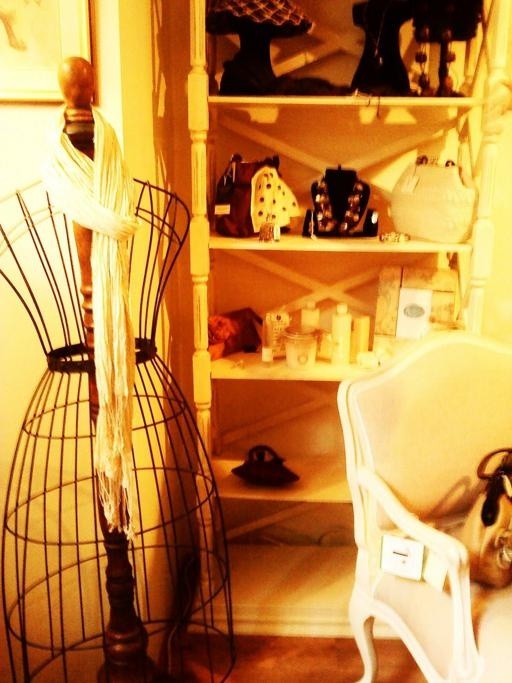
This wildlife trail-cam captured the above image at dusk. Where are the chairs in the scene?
[335,334,512,683]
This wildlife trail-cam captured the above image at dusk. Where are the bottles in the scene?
[301,300,319,329]
[332,304,351,367]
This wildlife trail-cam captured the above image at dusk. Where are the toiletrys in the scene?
[259,251,457,369]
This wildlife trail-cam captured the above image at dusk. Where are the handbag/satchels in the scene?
[462,447,512,588]
[390,155,477,243]
[209,153,290,242]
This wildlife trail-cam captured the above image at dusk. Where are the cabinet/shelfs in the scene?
[182,1,509,642]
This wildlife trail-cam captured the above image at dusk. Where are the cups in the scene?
[280,325,319,370]
